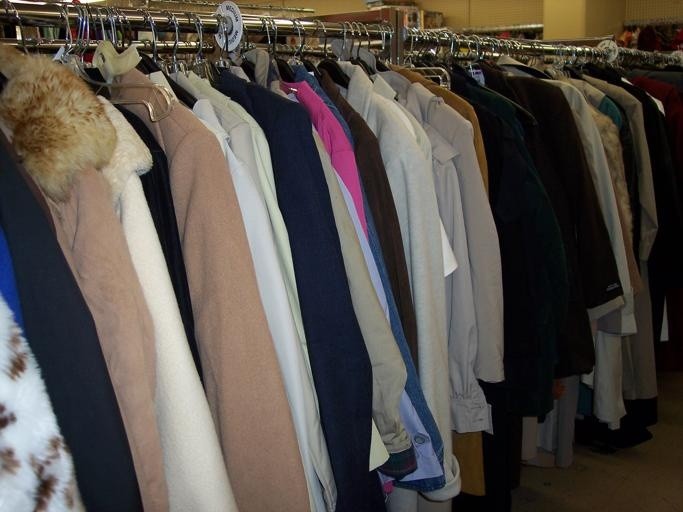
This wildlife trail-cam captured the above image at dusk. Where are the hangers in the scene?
[0,1,683,123]
